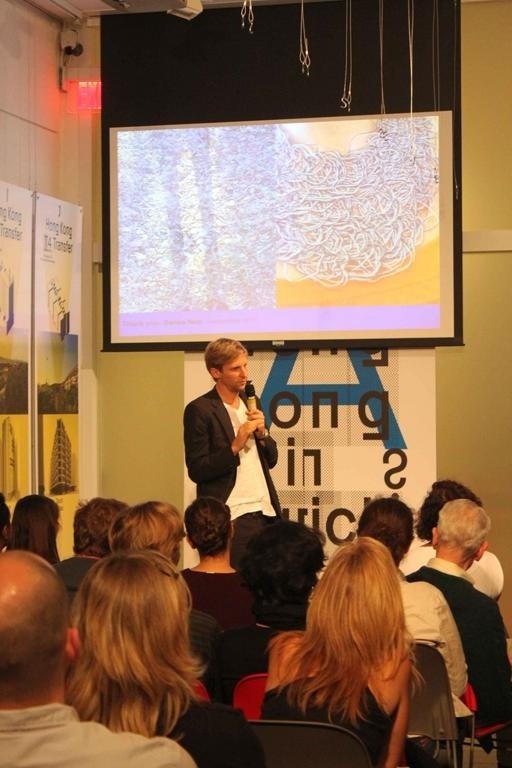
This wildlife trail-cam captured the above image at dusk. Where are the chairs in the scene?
[459,683,505,767]
[193,679,209,700]
[404,643,461,768]
[246,721,371,768]
[234,675,262,719]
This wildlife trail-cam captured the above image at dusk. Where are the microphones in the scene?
[245,384,257,412]
